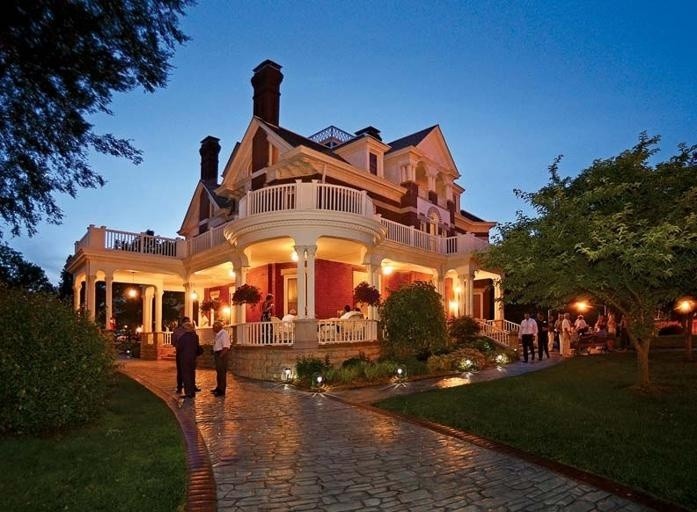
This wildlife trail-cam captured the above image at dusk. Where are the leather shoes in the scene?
[177,386,201,398]
[211,387,224,396]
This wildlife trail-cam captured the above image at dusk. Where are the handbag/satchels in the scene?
[196,343,204,355]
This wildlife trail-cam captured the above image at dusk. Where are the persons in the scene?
[260,294,297,344]
[337,304,363,343]
[134,319,177,340]
[518,311,628,363]
[171,316,231,398]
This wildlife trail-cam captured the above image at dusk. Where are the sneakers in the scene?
[523,353,535,362]
